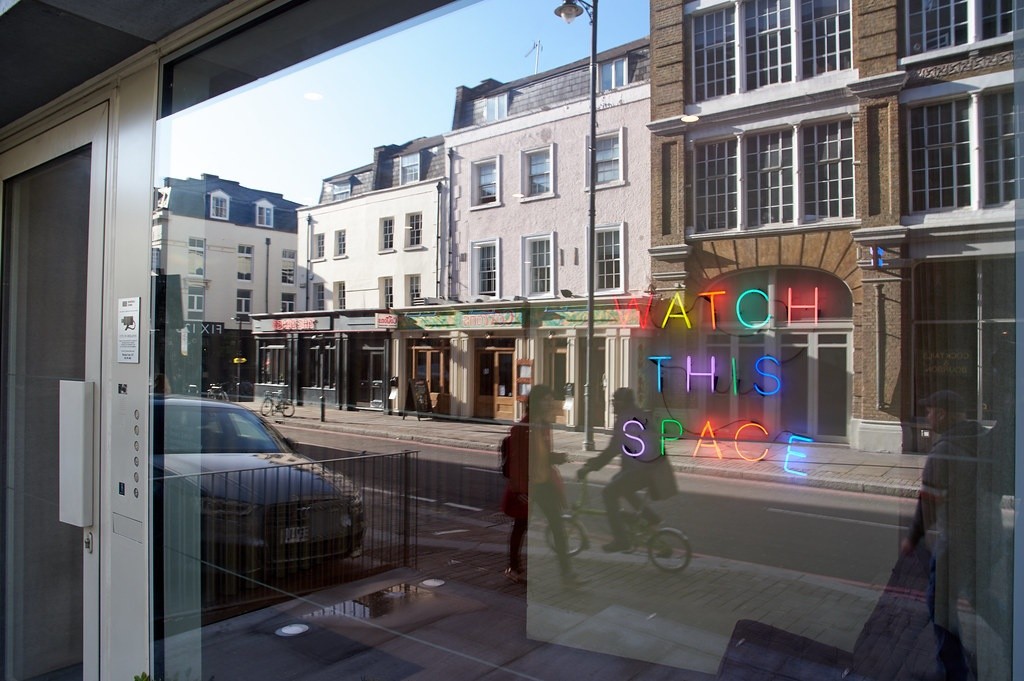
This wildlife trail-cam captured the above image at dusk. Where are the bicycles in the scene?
[261,391,296,417]
[207,384,230,402]
[546,470,692,572]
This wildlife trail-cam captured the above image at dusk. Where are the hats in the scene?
[916,390,964,414]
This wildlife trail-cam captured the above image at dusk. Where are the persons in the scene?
[504,385,590,586]
[580,388,678,552]
[901,391,1013,681]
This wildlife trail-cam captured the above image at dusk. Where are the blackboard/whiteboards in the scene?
[410,379,433,411]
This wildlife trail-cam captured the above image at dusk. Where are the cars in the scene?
[148,392,364,596]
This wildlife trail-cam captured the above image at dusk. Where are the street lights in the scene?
[554,0,598,452]
[230,317,241,390]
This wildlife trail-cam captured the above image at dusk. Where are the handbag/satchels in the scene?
[646,462,678,501]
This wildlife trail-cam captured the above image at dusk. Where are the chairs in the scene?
[713,491,1005,681]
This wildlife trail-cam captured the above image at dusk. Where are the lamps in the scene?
[560,290,572,298]
[422,333,429,339]
[548,330,555,339]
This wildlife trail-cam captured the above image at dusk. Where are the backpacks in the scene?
[500,436,511,477]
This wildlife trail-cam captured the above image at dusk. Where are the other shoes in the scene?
[648,516,659,527]
[506,568,527,587]
[602,540,630,552]
